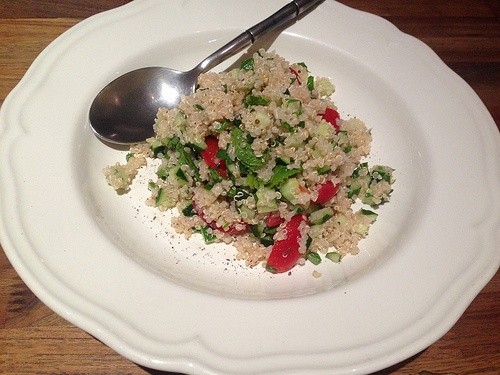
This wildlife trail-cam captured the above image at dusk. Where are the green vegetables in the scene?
[148,59,393,265]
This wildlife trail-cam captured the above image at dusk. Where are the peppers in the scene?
[265,213,311,274]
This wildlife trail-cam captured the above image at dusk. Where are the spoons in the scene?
[88,0,325,145]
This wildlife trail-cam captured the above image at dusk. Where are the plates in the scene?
[1,1,499,375]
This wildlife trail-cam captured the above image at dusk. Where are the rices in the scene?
[102,49,396,279]
